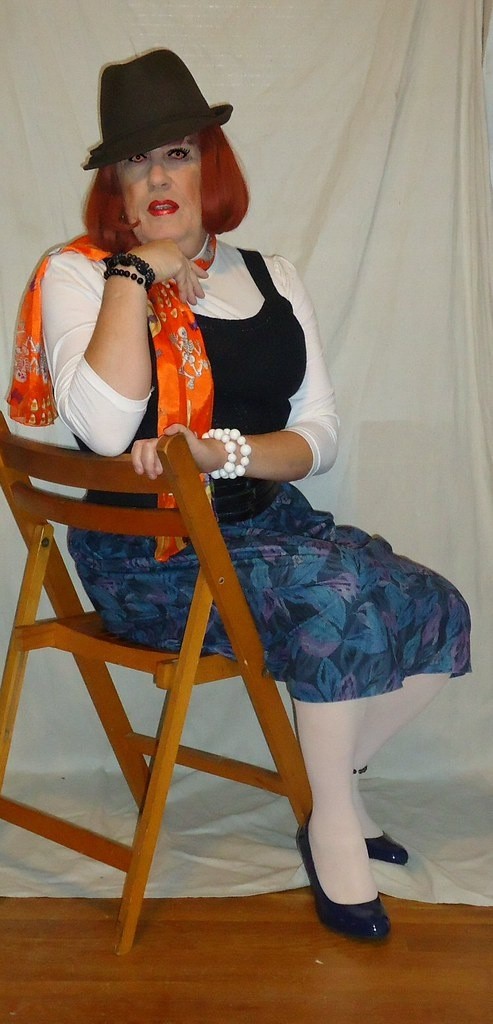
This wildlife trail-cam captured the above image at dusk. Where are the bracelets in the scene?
[104,253,155,290]
[202,428,251,479]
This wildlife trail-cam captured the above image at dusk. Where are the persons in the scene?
[7,50,472,942]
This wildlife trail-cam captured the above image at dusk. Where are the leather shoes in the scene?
[294,809,390,940]
[365,832,408,865]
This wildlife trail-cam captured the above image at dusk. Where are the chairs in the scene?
[0,410,312,956]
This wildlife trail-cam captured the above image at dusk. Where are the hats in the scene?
[82,49,233,171]
